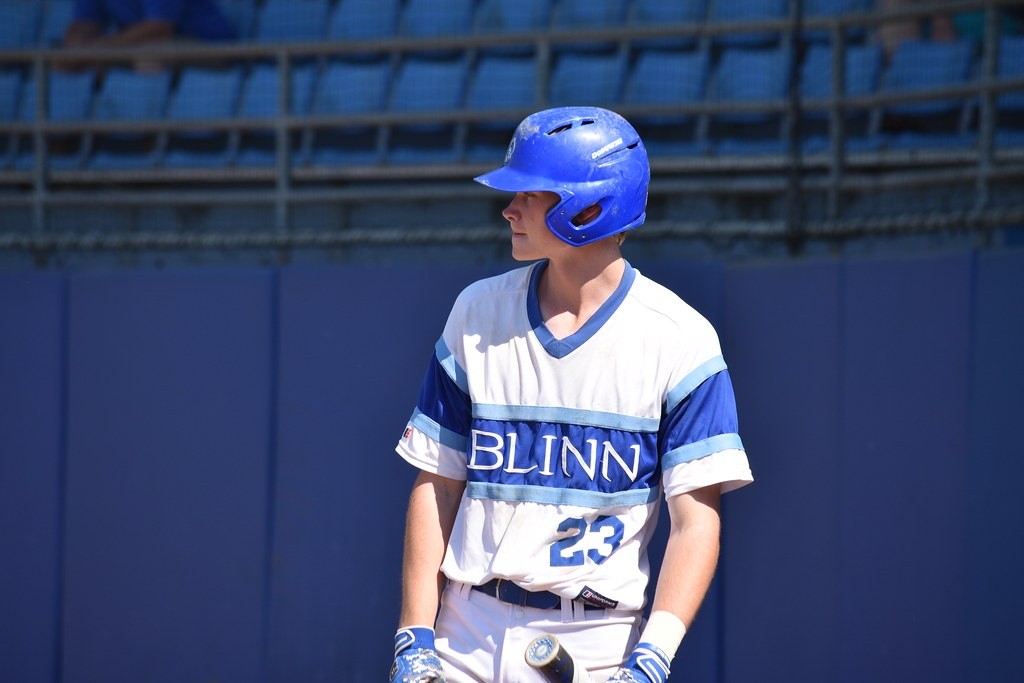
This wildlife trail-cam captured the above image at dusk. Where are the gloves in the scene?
[389,626,448,683]
[600,641,671,683]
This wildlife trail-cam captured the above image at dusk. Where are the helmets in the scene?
[474,107,651,247]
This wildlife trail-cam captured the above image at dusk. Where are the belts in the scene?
[471,577,608,610]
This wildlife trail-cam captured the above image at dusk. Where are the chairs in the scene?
[0,0,1024,182]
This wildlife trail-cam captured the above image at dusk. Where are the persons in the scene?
[48,0,241,72]
[390,105,753,681]
[870,3,1024,70]
